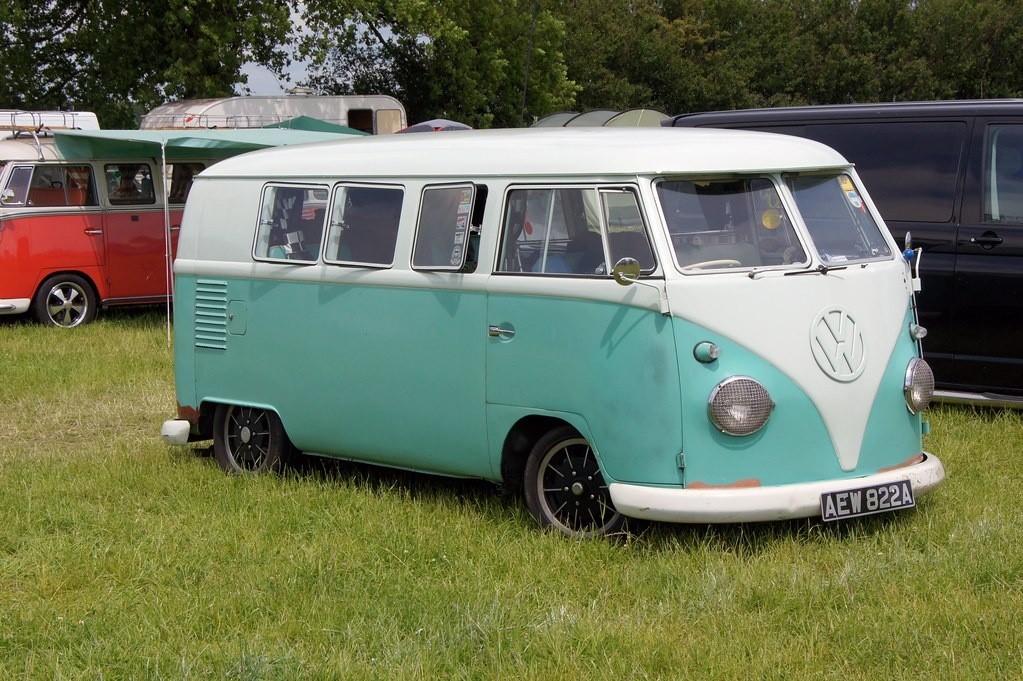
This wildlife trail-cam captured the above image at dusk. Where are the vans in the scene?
[160,126,947,545]
[135,87,409,210]
[660,97,1022,411]
[0,132,221,328]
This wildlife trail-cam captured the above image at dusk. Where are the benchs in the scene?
[7,187,86,206]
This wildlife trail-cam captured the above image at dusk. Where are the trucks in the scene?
[1,110,102,132]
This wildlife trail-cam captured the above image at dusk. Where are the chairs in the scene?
[676,244,764,269]
